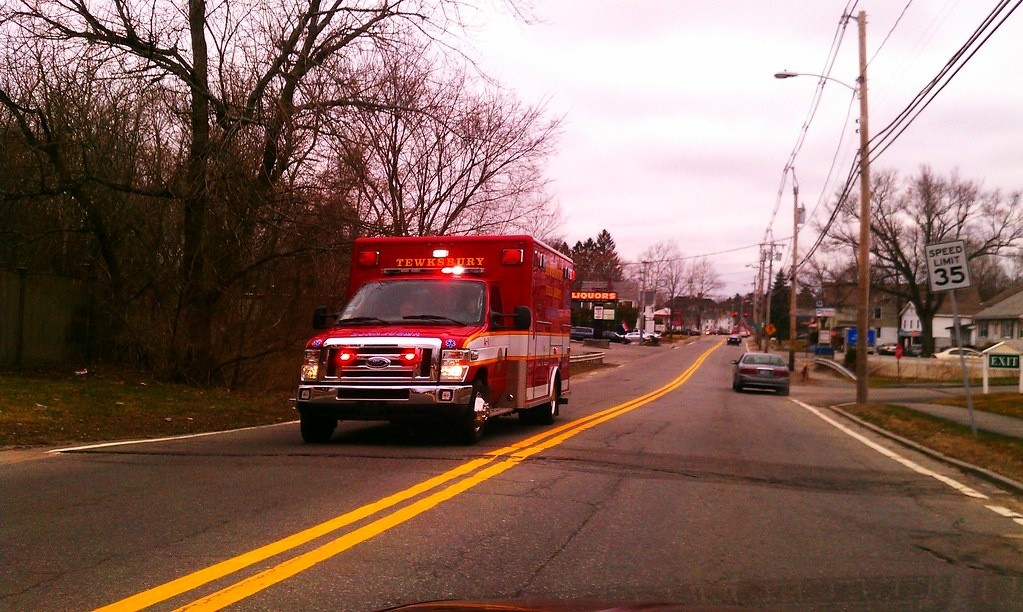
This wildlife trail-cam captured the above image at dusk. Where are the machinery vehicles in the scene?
[288,235,574,443]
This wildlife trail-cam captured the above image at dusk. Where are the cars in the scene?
[731,352,791,394]
[931,347,981,360]
[604,328,662,341]
[690,326,751,345]
[876,343,898,352]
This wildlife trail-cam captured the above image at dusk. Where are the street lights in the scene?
[774,72,870,402]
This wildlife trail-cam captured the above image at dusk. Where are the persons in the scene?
[398,299,415,316]
[458,287,480,321]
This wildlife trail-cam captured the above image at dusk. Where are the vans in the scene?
[570,325,594,340]
